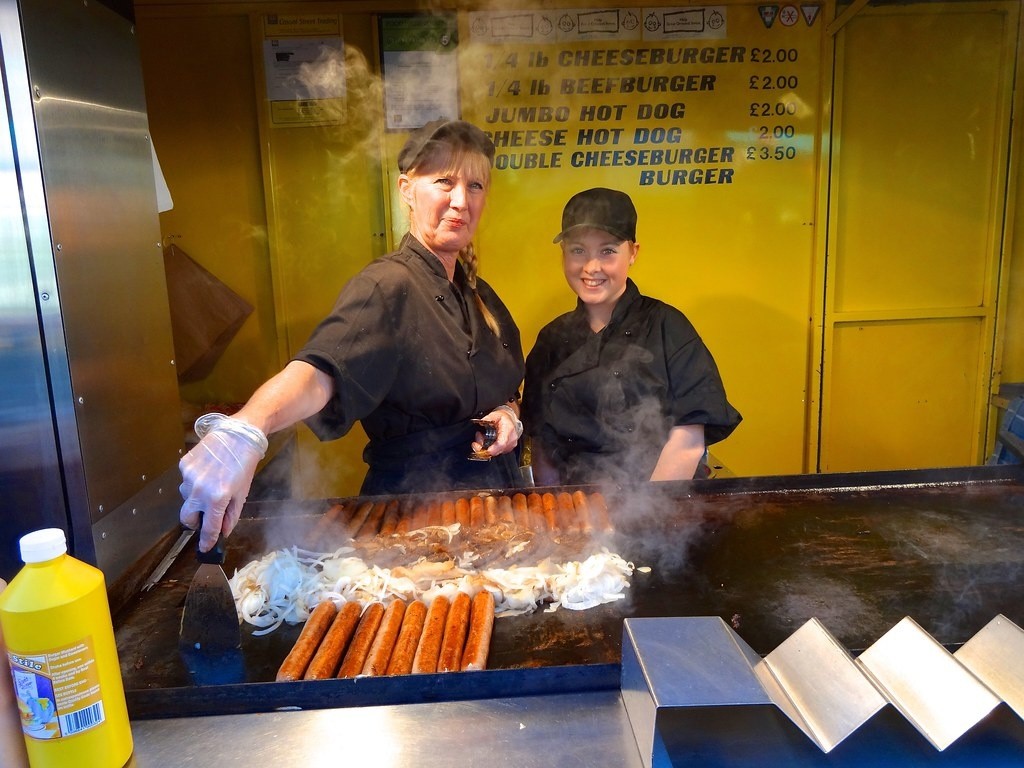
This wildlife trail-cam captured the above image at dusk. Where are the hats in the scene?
[552,188,637,244]
[396,119,495,175]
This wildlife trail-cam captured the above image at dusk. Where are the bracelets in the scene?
[498,405,523,439]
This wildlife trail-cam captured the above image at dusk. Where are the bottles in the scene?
[1,528,134,768]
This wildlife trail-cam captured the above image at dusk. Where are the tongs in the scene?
[467,417,499,462]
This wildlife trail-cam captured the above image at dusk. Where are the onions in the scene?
[223,542,652,635]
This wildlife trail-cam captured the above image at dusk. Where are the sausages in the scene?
[300,491,614,552]
[273,589,494,682]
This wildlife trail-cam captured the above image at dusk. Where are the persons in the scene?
[179,119,525,552]
[516,187,744,485]
